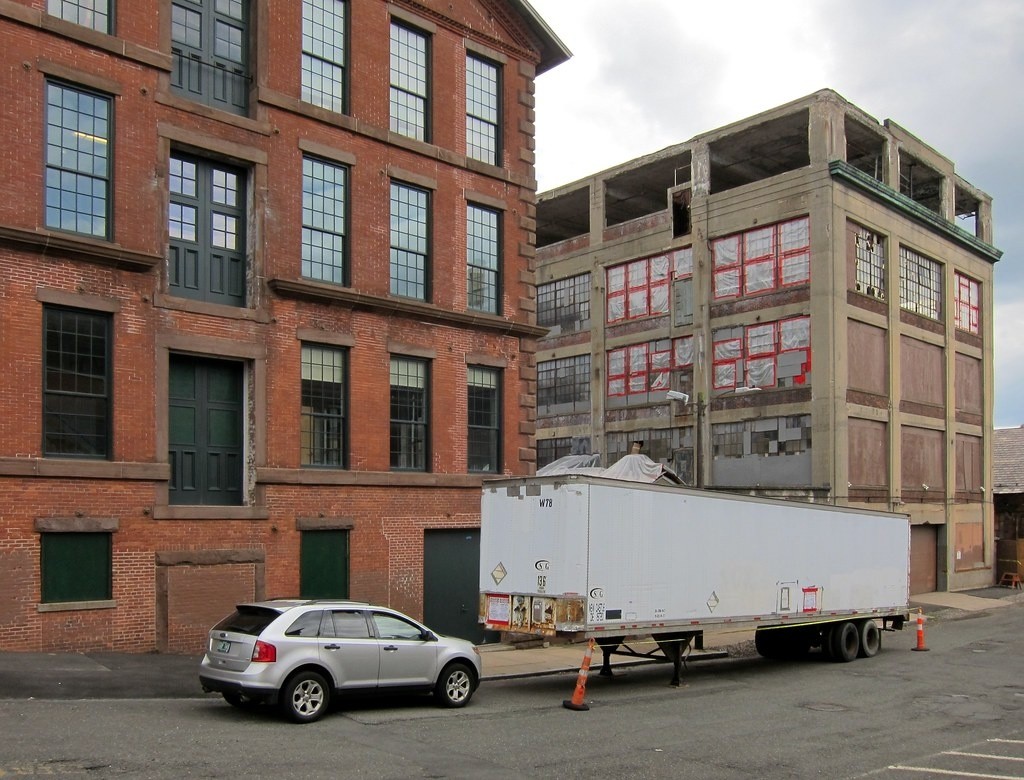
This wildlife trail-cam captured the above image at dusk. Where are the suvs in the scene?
[198,597,483,724]
